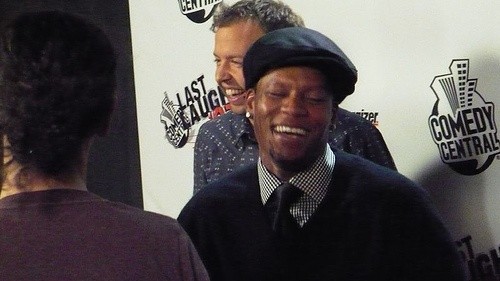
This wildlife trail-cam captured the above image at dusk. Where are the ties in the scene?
[268,181,304,238]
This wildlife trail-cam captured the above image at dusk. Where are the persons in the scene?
[177,26,470,281]
[192,0,398,197]
[0,10,211,281]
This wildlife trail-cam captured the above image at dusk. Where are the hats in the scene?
[242,27,358,106]
